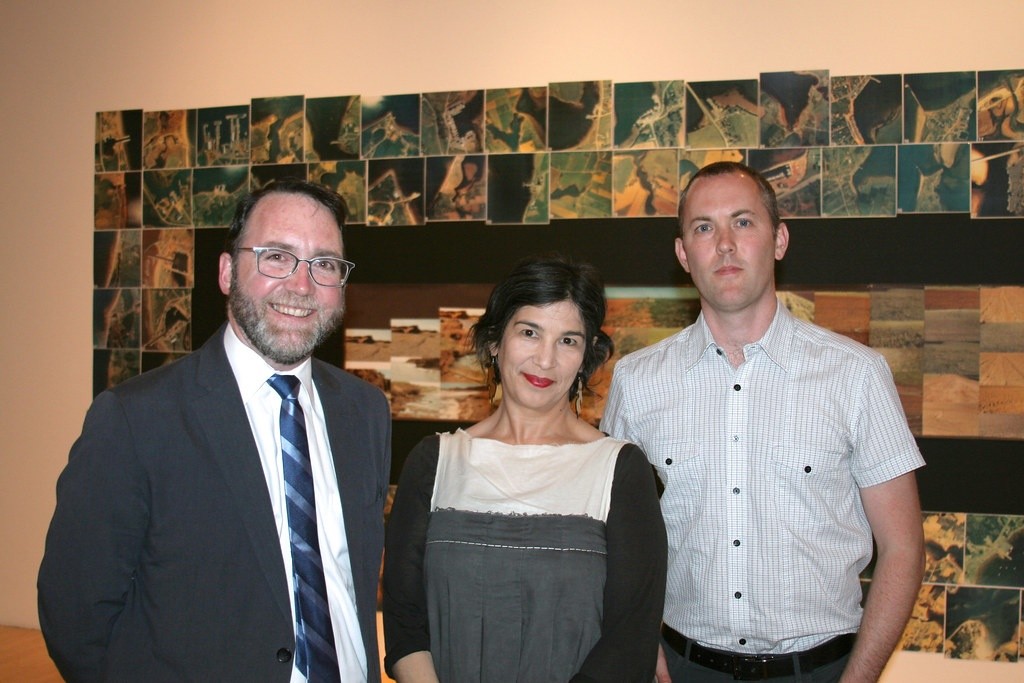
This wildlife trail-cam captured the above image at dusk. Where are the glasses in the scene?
[234,246,355,288]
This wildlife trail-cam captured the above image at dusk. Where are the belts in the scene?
[661,623,856,681]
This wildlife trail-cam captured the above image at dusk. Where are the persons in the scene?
[36,181,394,683]
[380,255,669,683]
[597,160,927,683]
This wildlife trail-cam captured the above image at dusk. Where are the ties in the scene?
[267,375,341,683]
